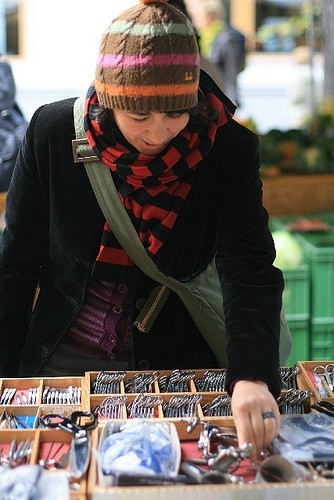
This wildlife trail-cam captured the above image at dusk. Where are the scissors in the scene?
[92,364,334,418]
[42,410,98,480]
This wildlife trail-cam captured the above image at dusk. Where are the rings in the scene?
[262,411,276,420]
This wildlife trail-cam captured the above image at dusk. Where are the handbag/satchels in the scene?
[176,248,293,369]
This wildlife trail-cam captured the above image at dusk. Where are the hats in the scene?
[95,0,199,111]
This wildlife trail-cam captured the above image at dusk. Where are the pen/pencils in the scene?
[0,385,81,429]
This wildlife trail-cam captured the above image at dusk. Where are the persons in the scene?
[0,0,282,467]
[199,2,249,111]
